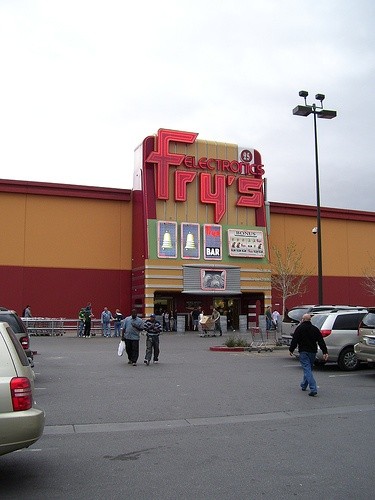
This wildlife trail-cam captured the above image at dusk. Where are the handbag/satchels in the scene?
[118,341,126,357]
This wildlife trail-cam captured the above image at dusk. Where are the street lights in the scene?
[291,91,338,307]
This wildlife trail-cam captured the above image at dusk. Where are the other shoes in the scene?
[133,363,135,366]
[144,360,149,365]
[154,359,158,363]
[308,392,317,396]
[128,360,131,364]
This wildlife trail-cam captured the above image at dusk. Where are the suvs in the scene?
[0,322,46,455]
[1,307,39,368]
[353,308,375,362]
[292,308,370,370]
[288,304,369,338]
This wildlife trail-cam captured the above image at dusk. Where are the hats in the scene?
[150,314,156,318]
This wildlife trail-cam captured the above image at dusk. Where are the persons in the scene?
[211,307,223,336]
[22,304,32,318]
[265,306,274,330]
[271,310,281,330]
[82,303,94,338]
[112,309,123,337]
[100,307,115,338]
[121,309,145,366]
[77,307,87,337]
[158,306,203,332]
[217,307,237,333]
[143,314,163,365]
[289,314,328,396]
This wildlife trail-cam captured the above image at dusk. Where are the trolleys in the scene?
[199,321,218,338]
[247,326,278,353]
